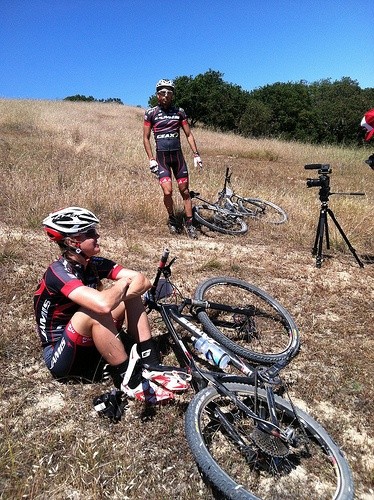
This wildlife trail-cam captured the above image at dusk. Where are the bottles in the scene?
[190,336,230,369]
[224,202,234,211]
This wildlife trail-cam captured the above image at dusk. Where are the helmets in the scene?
[43,206,98,242]
[156,78,175,89]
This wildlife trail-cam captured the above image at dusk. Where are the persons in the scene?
[33,206,189,403]
[143,79,203,241]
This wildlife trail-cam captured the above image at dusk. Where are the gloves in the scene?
[94,390,130,424]
[150,160,160,177]
[193,152,202,168]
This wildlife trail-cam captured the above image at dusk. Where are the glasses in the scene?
[156,89,173,95]
[81,229,96,238]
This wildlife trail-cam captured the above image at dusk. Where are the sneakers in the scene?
[144,366,190,392]
[168,221,177,235]
[121,379,175,402]
[182,221,198,240]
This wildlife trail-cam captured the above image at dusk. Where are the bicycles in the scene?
[138,248,354,500]
[188,167,288,234]
